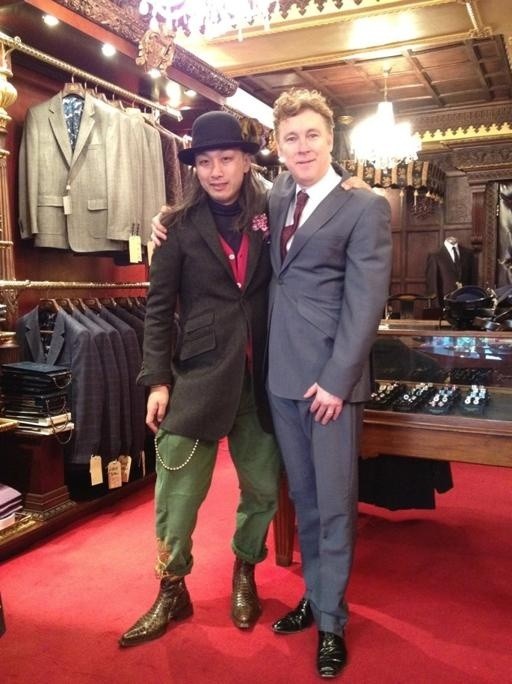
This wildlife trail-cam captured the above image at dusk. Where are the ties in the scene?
[451,246,458,261]
[281,191,308,262]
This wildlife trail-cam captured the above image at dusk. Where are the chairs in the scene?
[384,288,435,320]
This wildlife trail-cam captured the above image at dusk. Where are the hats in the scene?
[178,111,260,165]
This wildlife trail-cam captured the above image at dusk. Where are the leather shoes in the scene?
[272,600,314,634]
[121,579,194,646]
[231,556,262,628]
[318,628,345,676]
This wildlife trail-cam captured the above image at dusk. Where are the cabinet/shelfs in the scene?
[1,328,36,542]
[273,315,512,571]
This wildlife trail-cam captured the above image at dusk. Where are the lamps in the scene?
[344,63,424,170]
[135,0,275,46]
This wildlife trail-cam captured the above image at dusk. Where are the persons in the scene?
[266,83,393,680]
[117,110,283,649]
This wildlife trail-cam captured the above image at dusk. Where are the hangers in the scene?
[28,280,154,312]
[53,72,179,129]
